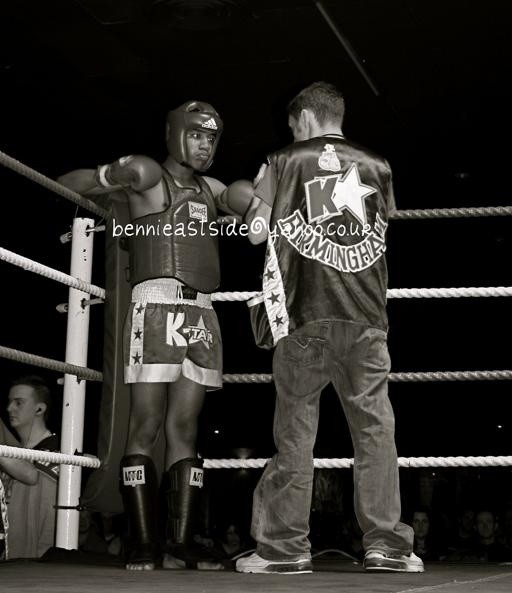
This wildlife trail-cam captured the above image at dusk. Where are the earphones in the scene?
[36,408,41,412]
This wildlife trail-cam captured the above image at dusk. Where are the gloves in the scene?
[217,180,255,217]
[94,154,163,191]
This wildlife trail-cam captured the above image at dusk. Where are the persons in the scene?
[233,80,426,577]
[56,99,253,573]
[1,374,512,559]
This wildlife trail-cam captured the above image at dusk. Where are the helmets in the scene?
[165,101,224,171]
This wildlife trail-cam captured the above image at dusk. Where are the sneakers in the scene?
[362,548,425,573]
[236,550,313,575]
